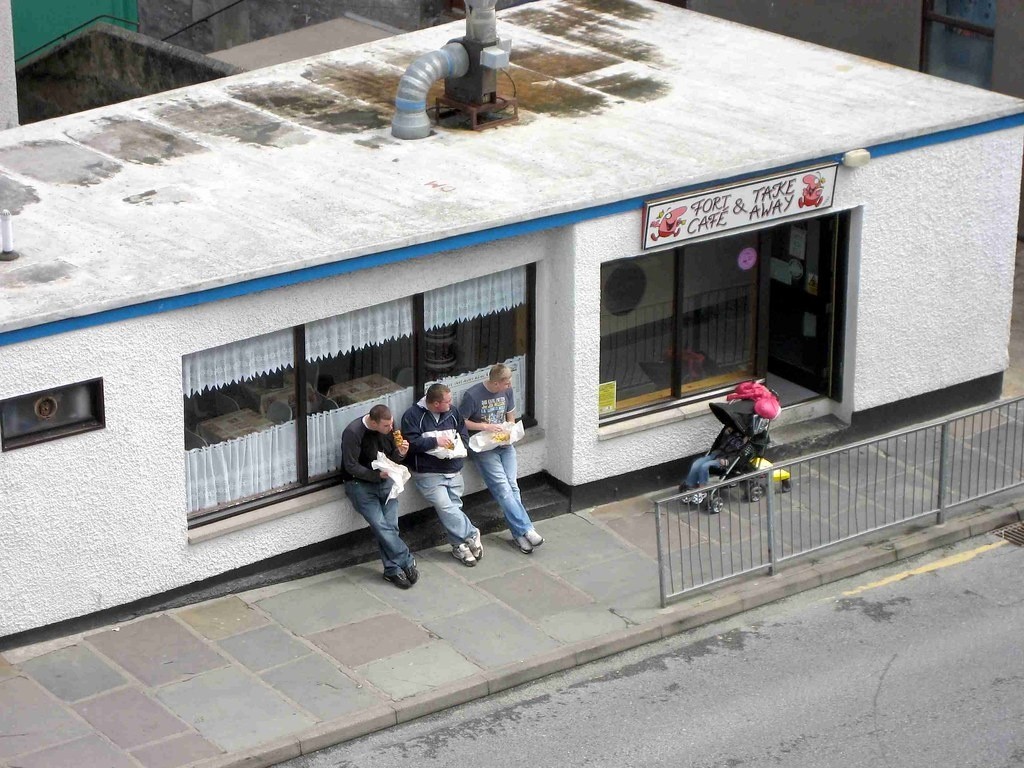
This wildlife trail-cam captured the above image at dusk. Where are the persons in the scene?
[401,384,484,567]
[682,428,747,505]
[341,404,420,590]
[459,364,545,554]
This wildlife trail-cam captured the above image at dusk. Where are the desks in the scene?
[239,372,319,416]
[638,352,727,389]
[196,406,275,443]
[328,373,404,405]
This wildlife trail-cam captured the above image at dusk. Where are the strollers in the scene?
[640,344,716,391]
[679,378,780,515]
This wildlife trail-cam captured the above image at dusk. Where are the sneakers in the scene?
[681,488,707,505]
[452,543,478,567]
[464,527,484,561]
[383,558,420,589]
[513,529,545,554]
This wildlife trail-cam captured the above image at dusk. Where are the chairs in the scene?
[188,391,240,419]
[398,367,413,389]
[310,362,340,409]
[266,401,293,424]
[185,426,208,449]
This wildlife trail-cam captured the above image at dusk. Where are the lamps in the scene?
[842,148,871,166]
